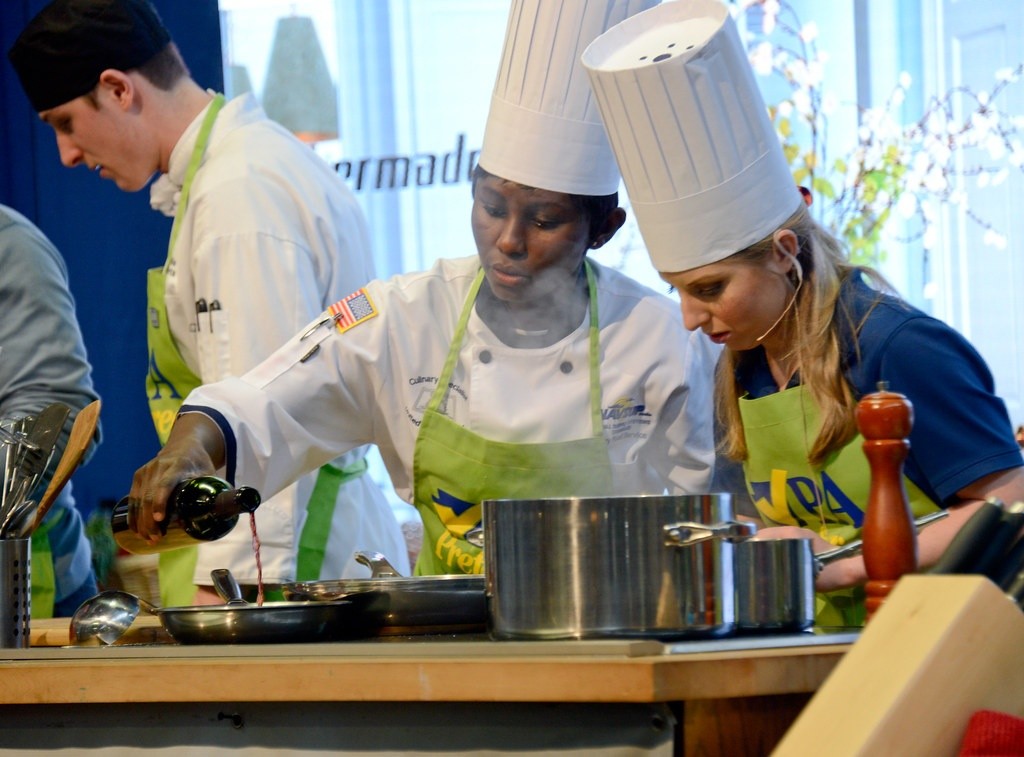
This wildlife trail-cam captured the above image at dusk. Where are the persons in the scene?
[581,0,1024,633]
[0,204,102,619]
[127,1,726,578]
[7,0,412,606]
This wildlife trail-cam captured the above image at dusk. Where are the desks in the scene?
[0,643,858,757]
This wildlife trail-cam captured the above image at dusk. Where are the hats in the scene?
[476,1,660,196]
[581,0,805,274]
[8,0,173,114]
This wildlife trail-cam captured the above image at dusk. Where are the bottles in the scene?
[111,476,261,557]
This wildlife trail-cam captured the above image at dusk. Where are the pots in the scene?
[735,510,950,633]
[482,491,756,638]
[285,550,485,625]
[153,568,354,643]
